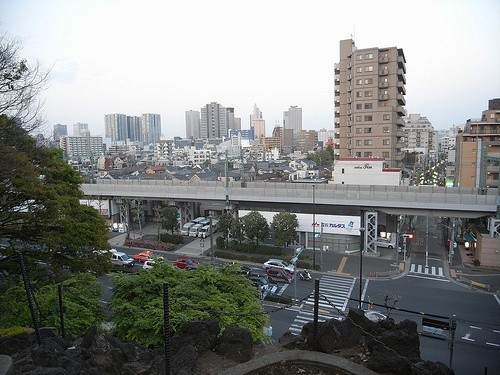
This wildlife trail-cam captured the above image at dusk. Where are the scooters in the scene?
[299,270,312,282]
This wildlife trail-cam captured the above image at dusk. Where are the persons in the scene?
[258,281,274,300]
[302,268,308,278]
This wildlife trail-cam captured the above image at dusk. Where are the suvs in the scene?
[109,252,135,270]
[376,238,396,249]
[263,258,296,274]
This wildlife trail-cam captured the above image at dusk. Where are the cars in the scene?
[143,261,156,270]
[92,247,117,257]
[240,266,272,287]
[175,256,201,270]
[134,251,158,263]
[267,268,293,285]
[364,310,386,322]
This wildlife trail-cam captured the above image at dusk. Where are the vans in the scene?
[183,217,220,238]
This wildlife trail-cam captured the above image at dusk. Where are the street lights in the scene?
[359,227,364,310]
[315,233,325,270]
